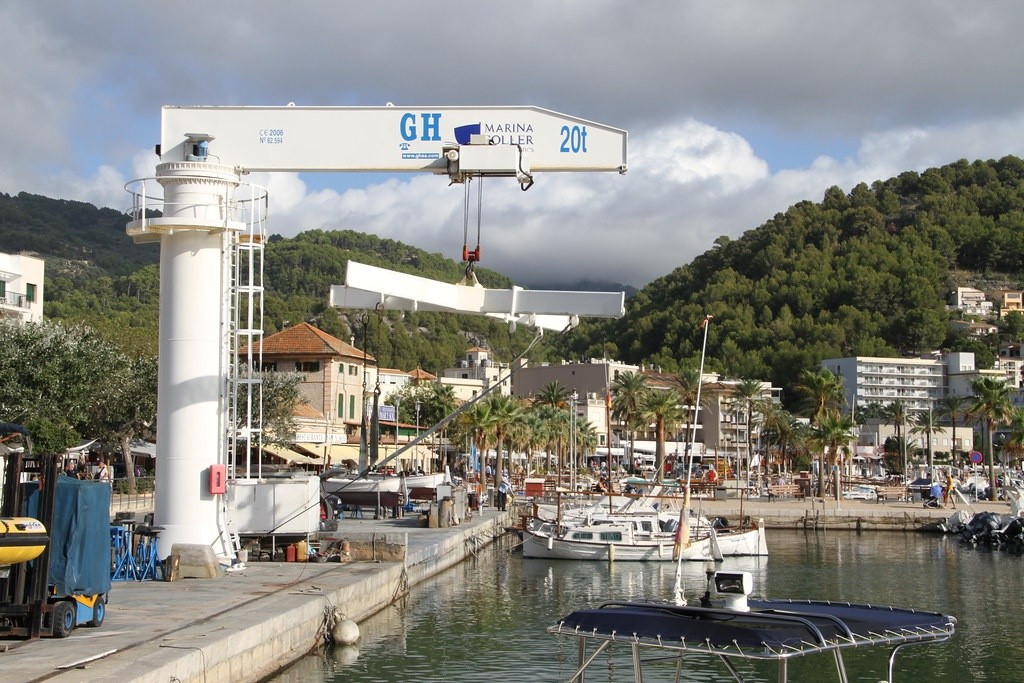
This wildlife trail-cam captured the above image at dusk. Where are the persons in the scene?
[341,459,426,519]
[944,471,956,509]
[589,455,646,477]
[595,478,615,494]
[485,463,511,512]
[66,455,110,484]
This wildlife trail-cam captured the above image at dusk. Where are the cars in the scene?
[321,489,345,517]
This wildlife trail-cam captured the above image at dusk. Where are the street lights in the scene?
[393,395,404,473]
[415,399,422,475]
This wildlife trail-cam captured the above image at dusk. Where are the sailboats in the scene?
[546,314,959,683]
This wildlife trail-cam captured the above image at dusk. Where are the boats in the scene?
[320,300,445,507]
[521,309,769,562]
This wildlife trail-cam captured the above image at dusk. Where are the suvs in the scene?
[110,463,146,487]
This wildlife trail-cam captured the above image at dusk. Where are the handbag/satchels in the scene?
[93,473,100,482]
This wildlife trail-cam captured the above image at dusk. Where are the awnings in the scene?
[247,440,440,466]
[856,452,883,461]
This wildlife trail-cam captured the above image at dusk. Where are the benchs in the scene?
[588,480,622,500]
[768,484,807,502]
[875,487,914,504]
[678,478,718,501]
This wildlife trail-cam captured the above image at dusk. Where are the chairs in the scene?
[543,479,558,493]
